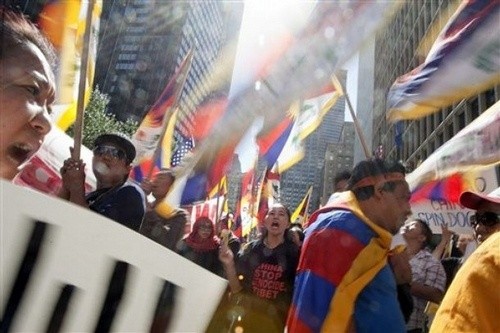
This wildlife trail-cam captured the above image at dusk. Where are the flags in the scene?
[21,0,500,256]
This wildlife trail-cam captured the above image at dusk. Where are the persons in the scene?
[0,13,499,332]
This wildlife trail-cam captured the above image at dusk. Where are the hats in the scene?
[460,187,500,210]
[94,132,136,164]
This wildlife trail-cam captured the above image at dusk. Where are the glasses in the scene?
[470,212,500,226]
[93,145,125,161]
